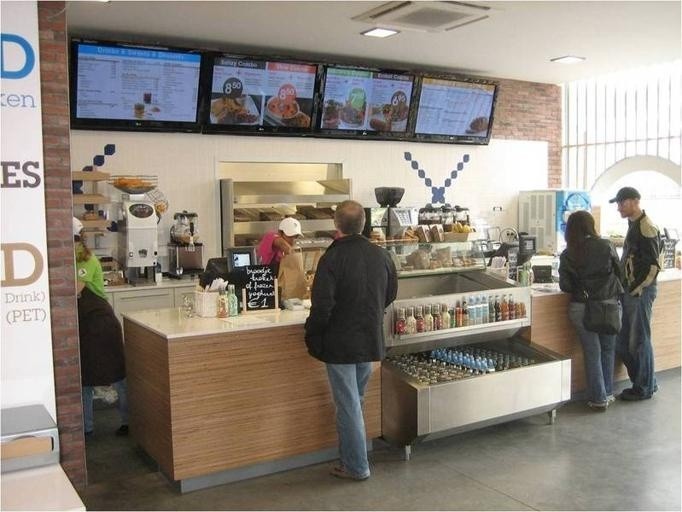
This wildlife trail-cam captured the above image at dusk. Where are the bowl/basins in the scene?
[375,187,405,208]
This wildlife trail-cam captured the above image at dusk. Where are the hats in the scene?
[73,215,84,237]
[609,187,641,203]
[278,217,305,238]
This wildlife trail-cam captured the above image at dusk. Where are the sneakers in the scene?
[330,463,370,481]
[586,400,607,412]
[622,384,659,393]
[116,425,130,435]
[619,391,652,400]
[606,393,615,405]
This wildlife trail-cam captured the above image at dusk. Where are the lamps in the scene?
[360,27,401,39]
[550,56,586,65]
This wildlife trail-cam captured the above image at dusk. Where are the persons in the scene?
[255,218,302,265]
[609,187,664,402]
[73,218,132,439]
[558,211,625,411]
[304,198,397,482]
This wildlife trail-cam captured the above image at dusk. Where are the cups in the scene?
[178,305,192,325]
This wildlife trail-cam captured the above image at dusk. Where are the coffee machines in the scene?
[167,209,205,279]
[106,175,159,287]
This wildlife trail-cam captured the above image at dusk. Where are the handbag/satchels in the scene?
[563,249,621,334]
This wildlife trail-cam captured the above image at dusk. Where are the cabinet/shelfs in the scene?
[383,238,572,461]
[221,176,353,256]
[531,268,681,392]
[121,274,383,495]
[105,274,198,323]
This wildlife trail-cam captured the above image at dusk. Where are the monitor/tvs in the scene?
[202,50,324,141]
[70,35,207,136]
[411,71,502,145]
[315,63,419,141]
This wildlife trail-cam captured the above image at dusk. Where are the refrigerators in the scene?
[518,189,592,275]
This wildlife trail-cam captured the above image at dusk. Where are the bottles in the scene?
[425,305,433,331]
[482,296,489,324]
[215,284,229,318]
[521,302,526,319]
[509,294,516,319]
[474,297,481,325]
[440,305,450,329]
[227,284,238,317]
[396,307,406,335]
[495,295,502,322]
[468,297,475,325]
[521,263,529,287]
[449,307,456,328]
[462,297,469,326]
[502,295,510,320]
[432,304,442,330]
[527,262,534,285]
[515,302,521,319]
[488,296,494,323]
[385,345,535,385]
[407,307,416,334]
[456,299,462,327]
[417,306,424,333]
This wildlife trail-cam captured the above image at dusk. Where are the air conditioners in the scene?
[352,0,492,36]
